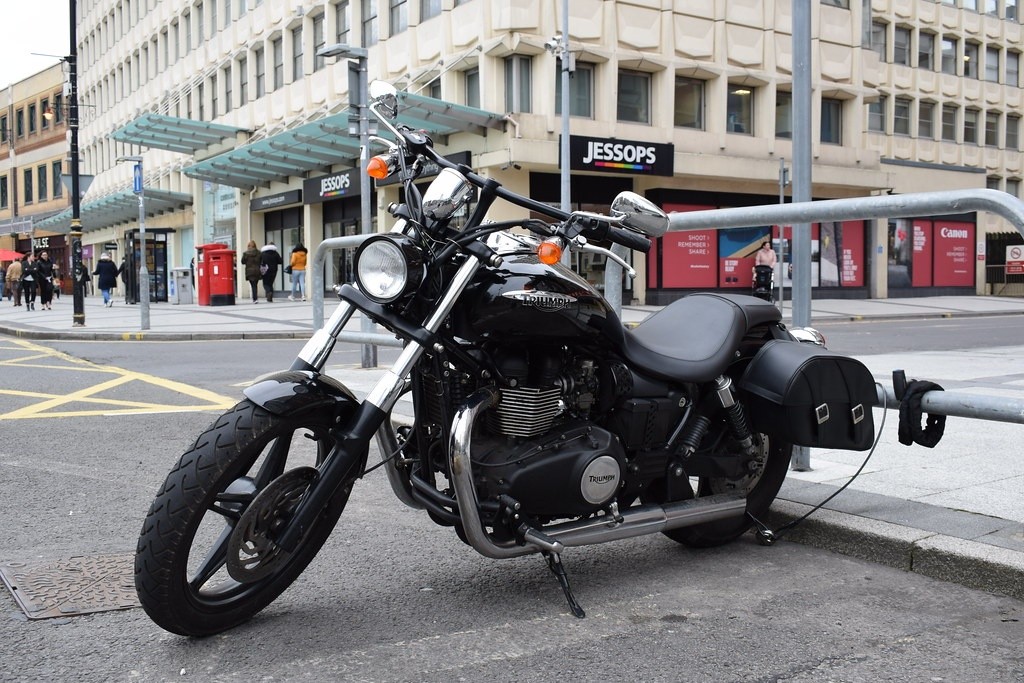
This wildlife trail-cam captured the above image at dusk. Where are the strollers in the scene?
[751,265,775,302]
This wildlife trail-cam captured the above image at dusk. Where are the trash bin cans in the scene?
[195,243,228,307]
[169,267,194,306]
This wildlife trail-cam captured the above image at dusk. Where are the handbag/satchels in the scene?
[259,263,269,276]
[283,265,292,275]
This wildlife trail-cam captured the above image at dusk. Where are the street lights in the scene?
[316,44,381,368]
[114,155,152,330]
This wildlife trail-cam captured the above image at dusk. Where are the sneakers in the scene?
[109,301,113,307]
[104,304,108,308]
[301,296,306,301]
[287,296,295,301]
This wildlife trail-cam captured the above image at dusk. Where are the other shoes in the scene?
[46,301,51,310]
[18,304,22,306]
[14,305,16,306]
[0,299,2,301]
[27,305,30,311]
[253,300,258,304]
[266,292,273,302]
[41,304,45,310]
[31,302,34,310]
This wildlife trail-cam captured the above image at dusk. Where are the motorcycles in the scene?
[134,83,882,638]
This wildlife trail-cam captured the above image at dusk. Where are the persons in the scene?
[241,240,282,304]
[0,250,63,311]
[288,242,307,301]
[91,253,126,307]
[82,263,91,297]
[755,241,776,268]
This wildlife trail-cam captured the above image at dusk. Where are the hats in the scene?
[101,253,110,260]
